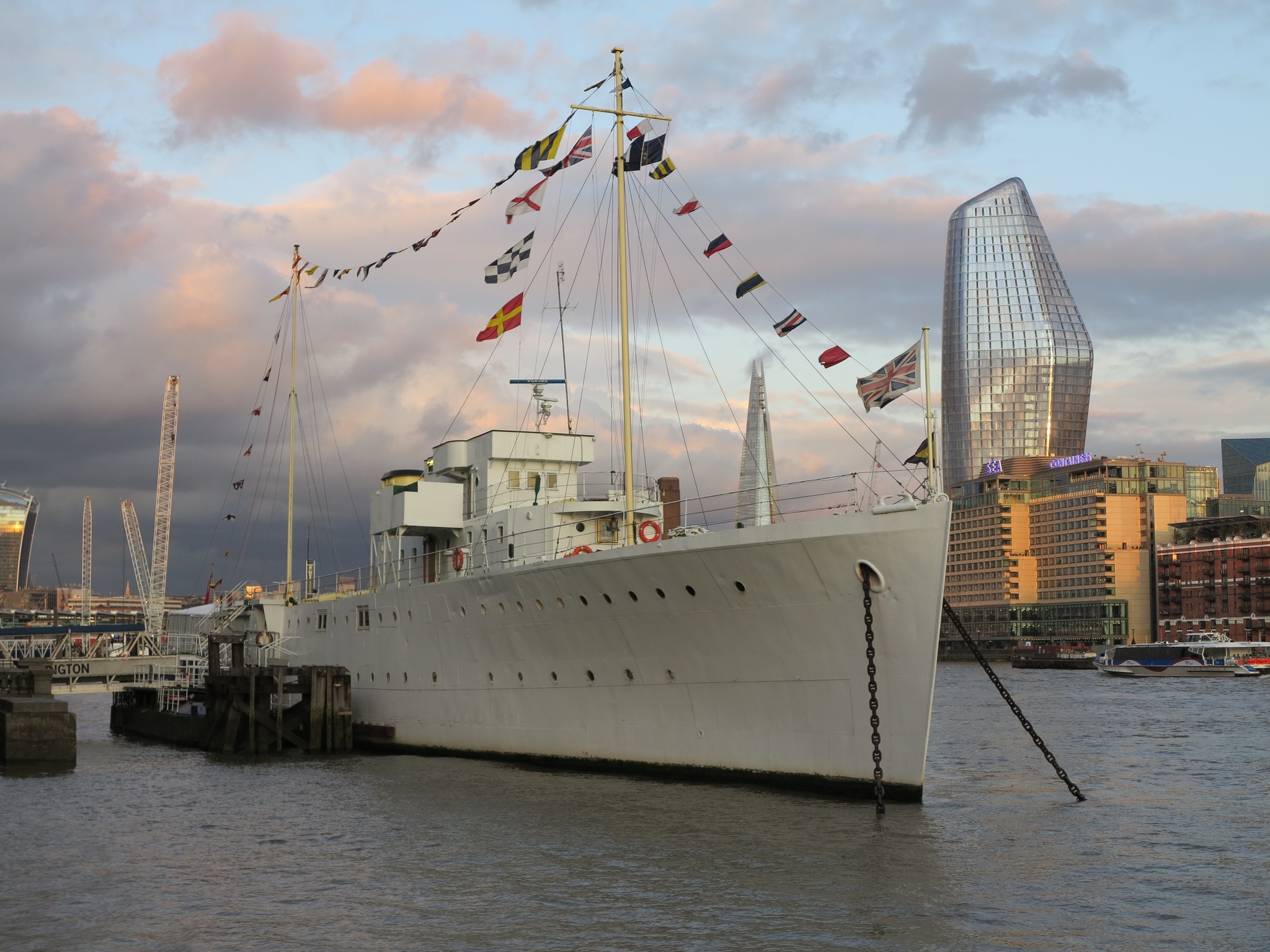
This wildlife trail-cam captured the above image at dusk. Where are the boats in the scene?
[1092,645,1261,678]
[161,45,957,805]
[1009,655,1099,669]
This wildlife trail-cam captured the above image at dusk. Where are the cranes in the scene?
[119,374,183,652]
[80,494,93,652]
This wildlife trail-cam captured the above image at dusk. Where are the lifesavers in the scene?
[565,554,573,558]
[256,632,273,649]
[167,697,186,701]
[1068,662,1073,669]
[1082,654,1085,657]
[453,548,464,571]
[573,546,593,555]
[1040,662,1045,668]
[1060,662,1065,668]
[1027,662,1031,668]
[639,521,660,543]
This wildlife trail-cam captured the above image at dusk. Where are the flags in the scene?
[856,339,921,413]
[902,431,936,469]
[204,63,851,605]
